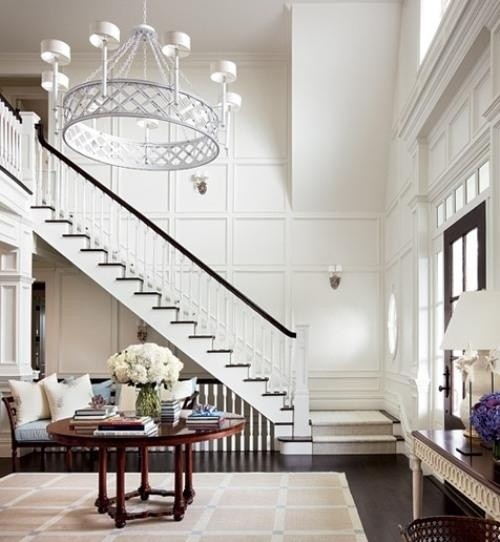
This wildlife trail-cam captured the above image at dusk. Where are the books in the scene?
[69,397,225,436]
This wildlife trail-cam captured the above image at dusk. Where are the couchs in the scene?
[1,379,198,464]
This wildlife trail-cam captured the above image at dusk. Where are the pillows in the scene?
[8,372,198,426]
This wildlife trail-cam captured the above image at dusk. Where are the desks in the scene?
[409,429,500,541]
[48,410,247,525]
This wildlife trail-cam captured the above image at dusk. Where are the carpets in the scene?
[0,471,368,540]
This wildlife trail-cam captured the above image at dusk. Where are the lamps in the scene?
[41,2,243,171]
[440,291,500,437]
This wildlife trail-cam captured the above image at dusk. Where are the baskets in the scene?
[398,515,500,542]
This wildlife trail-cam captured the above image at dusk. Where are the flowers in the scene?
[104,342,184,416]
[470,391,499,442]
